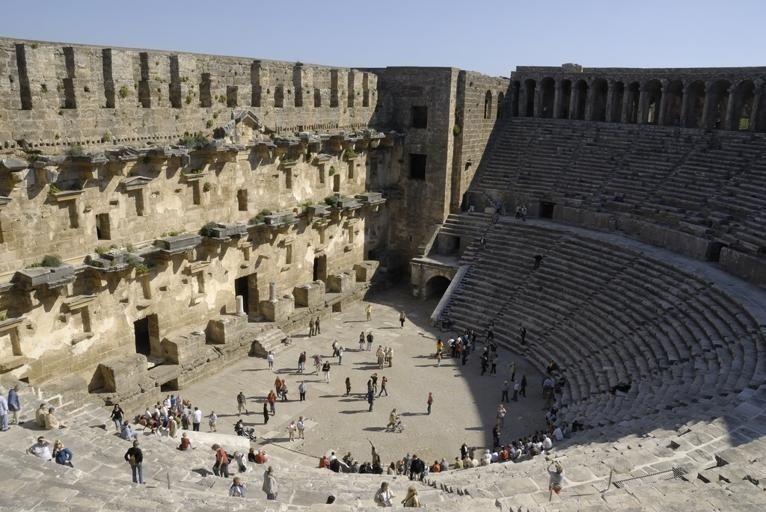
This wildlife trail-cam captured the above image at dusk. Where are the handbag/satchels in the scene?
[128,454,138,466]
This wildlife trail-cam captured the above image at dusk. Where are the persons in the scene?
[479,236,485,249]
[514,205,521,219]
[521,206,528,220]
[125,440,146,484]
[345,330,393,412]
[110,393,217,449]
[400,311,406,327]
[494,199,503,214]
[375,408,565,507]
[267,315,343,383]
[2,384,72,465]
[435,326,564,407]
[365,305,372,321]
[233,376,307,442]
[320,451,383,473]
[212,444,278,500]
[426,392,433,415]
[534,254,543,269]
